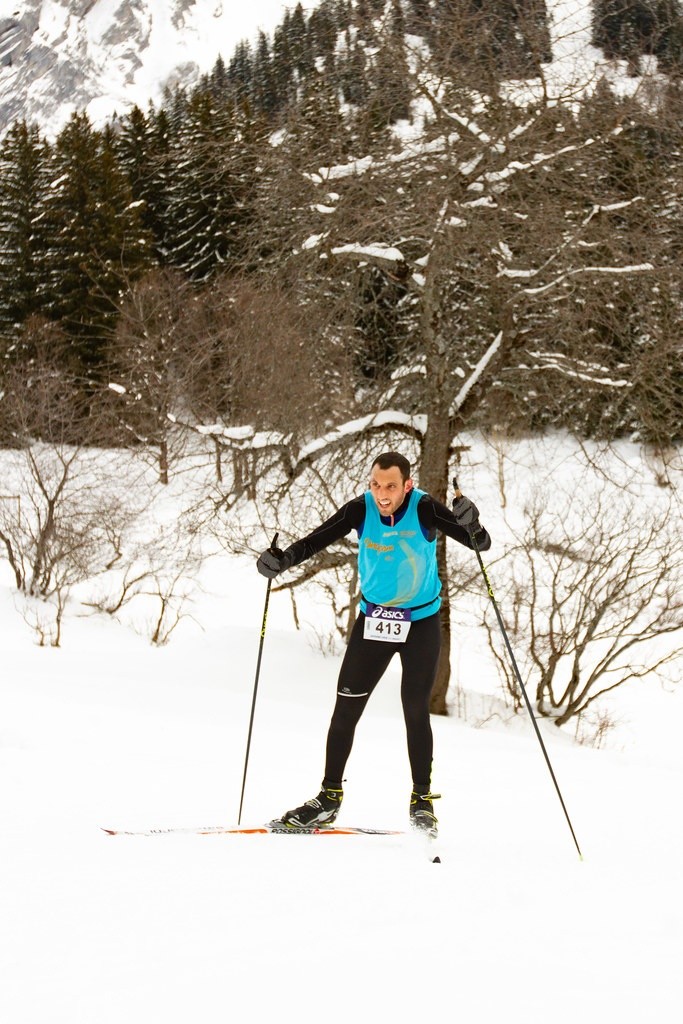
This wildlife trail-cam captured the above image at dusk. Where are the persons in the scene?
[256,452,491,831]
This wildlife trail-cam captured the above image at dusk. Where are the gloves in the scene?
[452,494,480,531]
[256,548,290,579]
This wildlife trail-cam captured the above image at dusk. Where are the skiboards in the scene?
[98,822,444,867]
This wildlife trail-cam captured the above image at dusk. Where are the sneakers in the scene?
[281,787,343,828]
[410,792,441,828]
